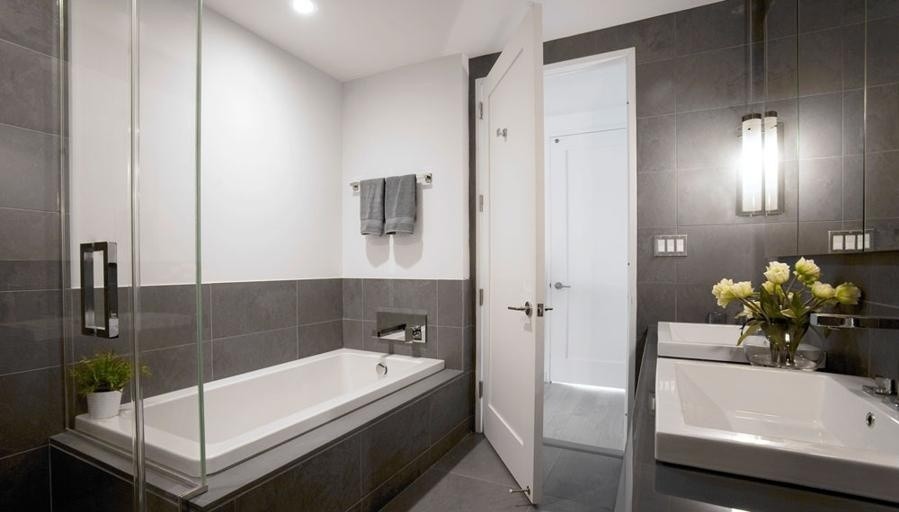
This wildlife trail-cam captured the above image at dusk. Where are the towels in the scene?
[359,178,385,237]
[385,174,417,234]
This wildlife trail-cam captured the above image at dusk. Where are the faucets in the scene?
[377,322,407,354]
[863,375,898,410]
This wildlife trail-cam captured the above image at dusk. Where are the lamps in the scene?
[764,0,782,216]
[740,0,765,217]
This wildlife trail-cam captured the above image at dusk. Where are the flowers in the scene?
[711,256,862,367]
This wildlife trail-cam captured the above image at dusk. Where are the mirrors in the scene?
[765,0,899,258]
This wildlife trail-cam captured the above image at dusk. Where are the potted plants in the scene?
[65,350,154,420]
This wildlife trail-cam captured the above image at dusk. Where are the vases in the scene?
[741,319,827,373]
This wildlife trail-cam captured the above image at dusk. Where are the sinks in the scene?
[654,357,899,503]
[657,320,821,362]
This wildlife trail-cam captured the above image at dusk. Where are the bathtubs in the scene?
[75,345,445,477]
